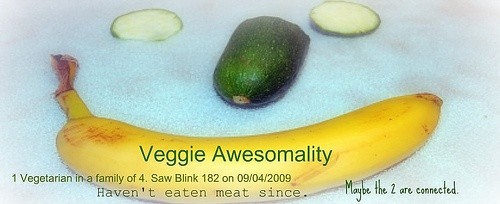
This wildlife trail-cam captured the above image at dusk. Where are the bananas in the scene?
[49,52,444,204]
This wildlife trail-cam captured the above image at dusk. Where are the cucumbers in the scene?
[210,15,311,110]
